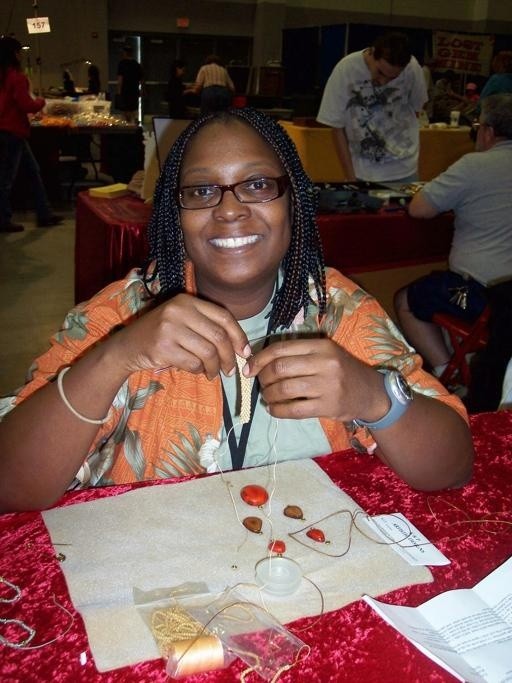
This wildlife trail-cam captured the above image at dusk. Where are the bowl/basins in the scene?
[318,189,350,211]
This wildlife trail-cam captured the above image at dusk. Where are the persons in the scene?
[165,62,196,118]
[0,37,64,232]
[115,46,144,111]
[0,107,473,515]
[315,33,430,184]
[394,93,512,399]
[191,55,236,113]
[435,51,512,115]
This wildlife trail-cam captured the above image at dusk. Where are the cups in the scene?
[368,188,390,212]
[99,92,106,102]
[450,111,460,126]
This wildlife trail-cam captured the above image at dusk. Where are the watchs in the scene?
[353,369,412,431]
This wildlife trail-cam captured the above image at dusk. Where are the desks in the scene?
[0,405,511,681]
[107,79,295,120]
[74,179,454,317]
[22,100,141,206]
[275,114,474,188]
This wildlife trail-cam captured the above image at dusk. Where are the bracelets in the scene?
[57,367,112,426]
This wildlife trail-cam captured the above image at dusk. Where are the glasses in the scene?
[174,173,291,211]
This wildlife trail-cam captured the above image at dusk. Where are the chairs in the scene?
[429,305,492,395]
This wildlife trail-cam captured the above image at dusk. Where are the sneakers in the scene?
[36,210,64,228]
[0,220,25,235]
